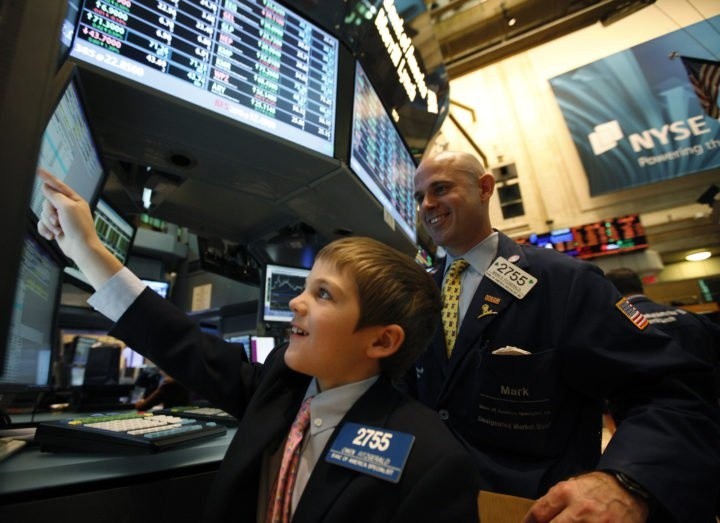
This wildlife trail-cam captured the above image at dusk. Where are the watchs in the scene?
[604,469,658,514]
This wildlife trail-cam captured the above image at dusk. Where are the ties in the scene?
[264,395,315,523]
[441,258,469,354]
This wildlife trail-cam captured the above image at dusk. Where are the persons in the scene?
[412,152,720,523]
[602,268,720,442]
[135,357,188,410]
[37,167,479,522]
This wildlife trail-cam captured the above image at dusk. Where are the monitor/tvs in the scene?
[0,1,423,397]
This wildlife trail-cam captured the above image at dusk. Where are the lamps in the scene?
[686,246,713,260]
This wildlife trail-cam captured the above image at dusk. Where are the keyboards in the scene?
[152,405,238,423]
[33,412,227,455]
